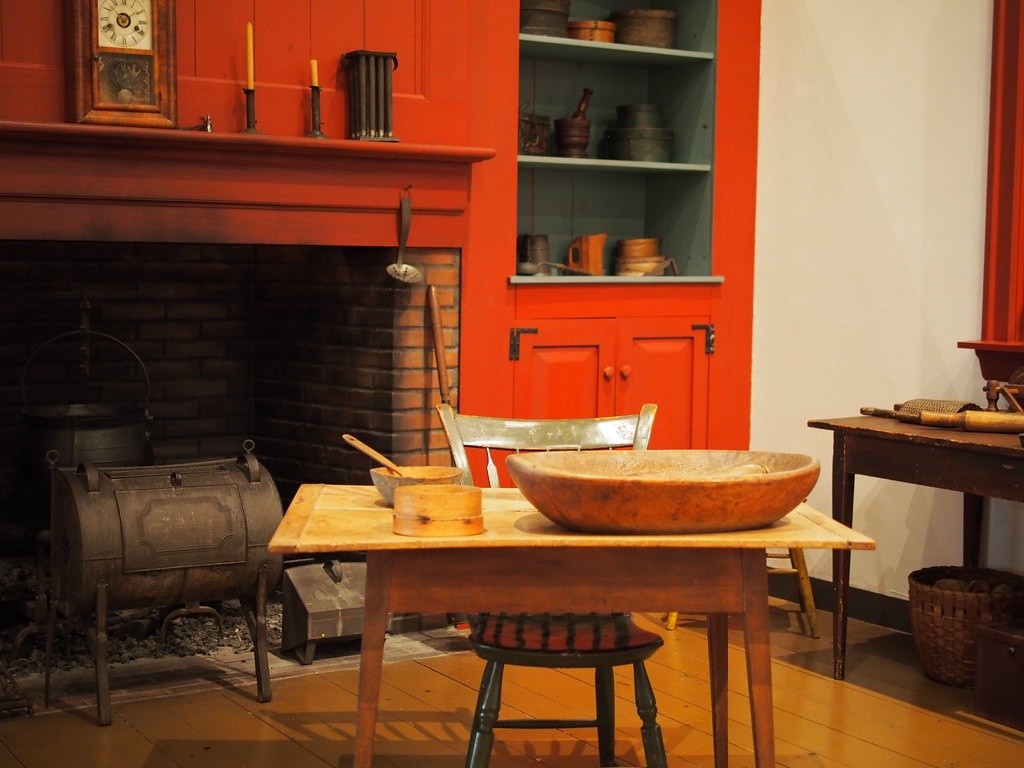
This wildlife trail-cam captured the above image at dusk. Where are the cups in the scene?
[523,233,549,275]
[567,232,608,275]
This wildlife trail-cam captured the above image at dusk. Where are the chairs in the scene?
[437,403,668,768]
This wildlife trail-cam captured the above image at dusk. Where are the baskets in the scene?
[908,566,1024,688]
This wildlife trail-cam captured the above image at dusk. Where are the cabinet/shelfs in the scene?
[459,0,762,487]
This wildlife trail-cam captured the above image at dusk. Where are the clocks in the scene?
[62,0,181,129]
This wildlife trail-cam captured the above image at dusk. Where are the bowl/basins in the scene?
[370,466,464,505]
[505,449,820,533]
[519,0,678,50]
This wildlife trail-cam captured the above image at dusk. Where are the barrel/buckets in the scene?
[17,327,152,487]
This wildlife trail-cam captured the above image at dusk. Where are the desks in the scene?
[268,484,876,768]
[808,409,1024,680]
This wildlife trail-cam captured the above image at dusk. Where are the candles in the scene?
[247,22,254,89]
[309,60,319,85]
